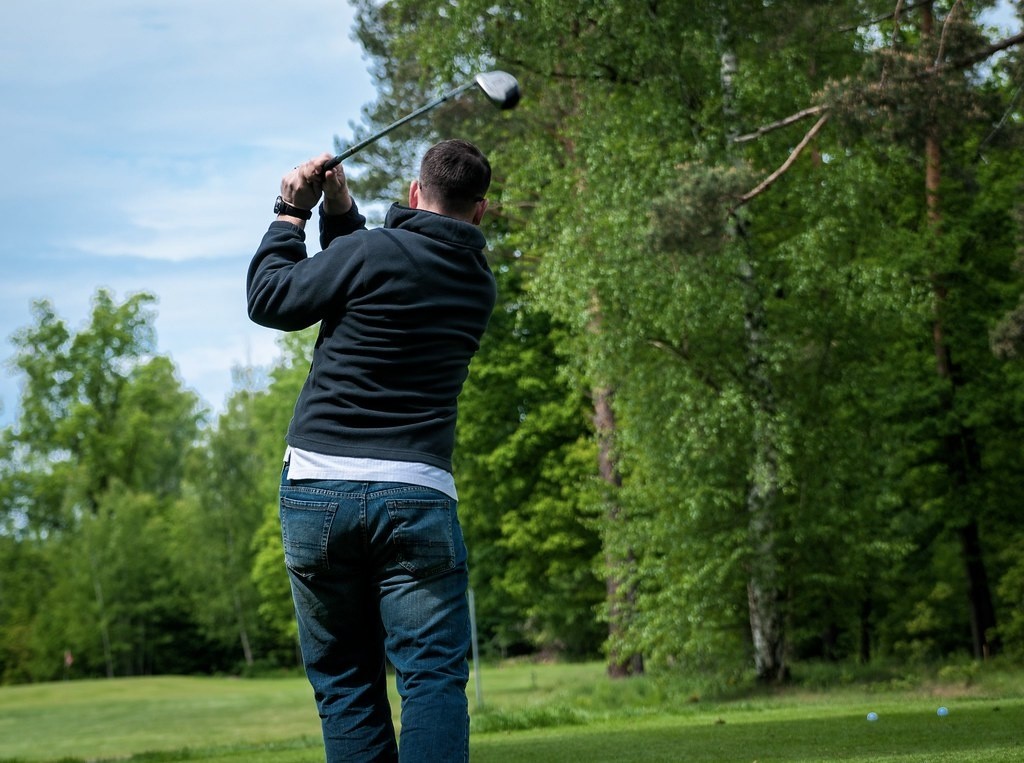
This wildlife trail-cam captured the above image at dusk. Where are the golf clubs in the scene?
[321,69,522,174]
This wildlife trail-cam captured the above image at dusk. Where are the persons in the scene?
[246,139,498,763]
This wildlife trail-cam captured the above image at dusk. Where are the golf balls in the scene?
[937,707,948,716]
[867,712,879,722]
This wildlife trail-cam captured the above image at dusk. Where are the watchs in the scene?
[273,195,313,220]
